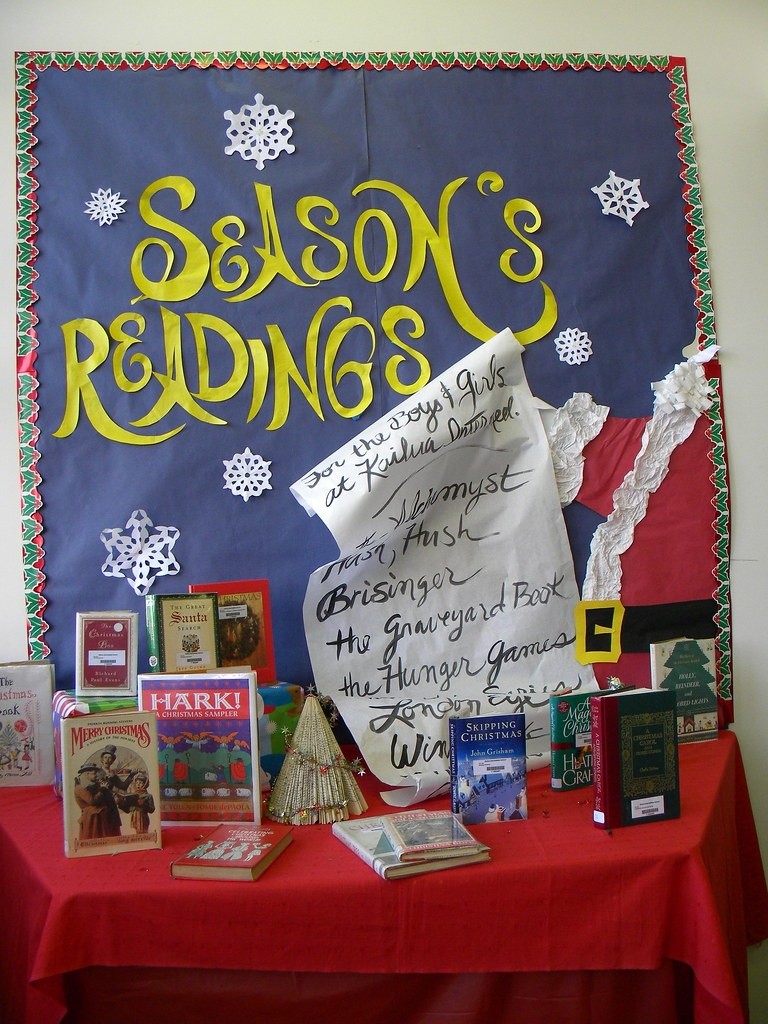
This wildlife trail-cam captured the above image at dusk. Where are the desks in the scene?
[1,728,768,1024]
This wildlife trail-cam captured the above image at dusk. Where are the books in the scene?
[59,707,163,856]
[649,635,719,743]
[136,665,263,827]
[188,577,277,685]
[376,810,482,861]
[168,821,294,883]
[449,710,529,825]
[143,593,222,673]
[2,660,55,787]
[330,808,492,881]
[589,684,682,828]
[76,611,138,697]
[550,683,612,790]
